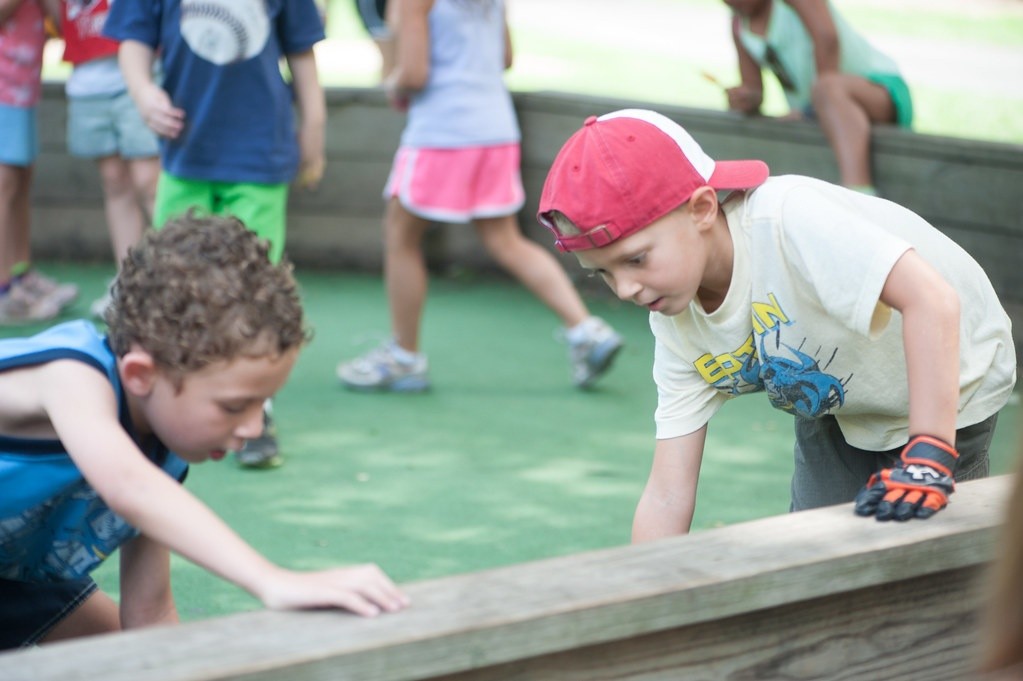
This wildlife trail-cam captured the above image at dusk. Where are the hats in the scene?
[536,109,770,253]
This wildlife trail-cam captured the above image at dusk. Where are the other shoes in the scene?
[0,279,60,323]
[9,260,78,308]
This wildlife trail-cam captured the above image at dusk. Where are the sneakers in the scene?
[334,336,430,390]
[233,398,282,468]
[564,315,624,386]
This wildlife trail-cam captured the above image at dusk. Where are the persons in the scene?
[336,0,624,394]
[60,0,162,268]
[0,205,408,655]
[724,0,915,199]
[0,0,77,327]
[535,107,1019,544]
[101,0,329,265]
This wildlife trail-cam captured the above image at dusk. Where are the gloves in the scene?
[855,433,961,521]
